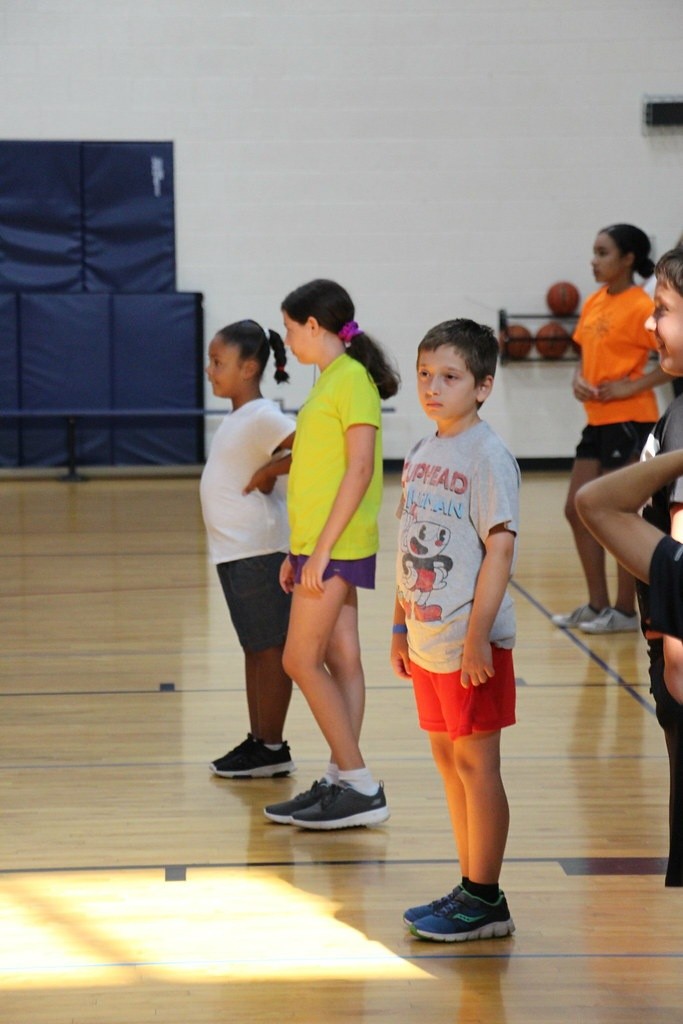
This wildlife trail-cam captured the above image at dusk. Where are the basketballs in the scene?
[546,282,580,313]
[570,328,582,356]
[534,324,569,357]
[498,325,533,356]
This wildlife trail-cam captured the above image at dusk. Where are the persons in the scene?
[552,224,683,888]
[391,319,522,943]
[200,279,400,829]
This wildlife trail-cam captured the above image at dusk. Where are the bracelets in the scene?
[392,624,407,633]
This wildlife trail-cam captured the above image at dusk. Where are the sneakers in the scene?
[551,605,597,628]
[264,777,389,829]
[403,883,516,942]
[579,608,640,633]
[210,734,298,779]
